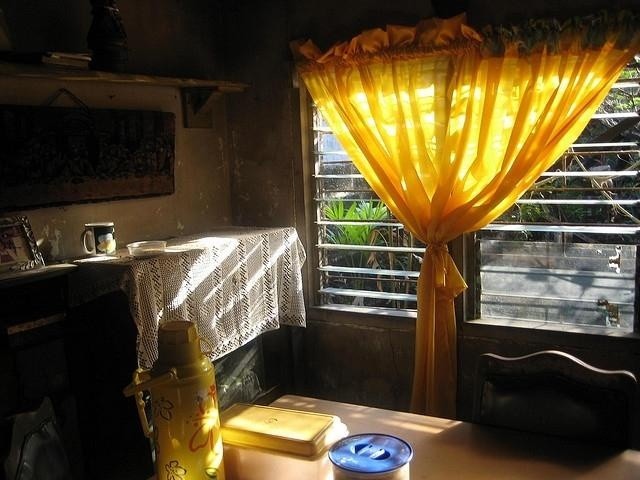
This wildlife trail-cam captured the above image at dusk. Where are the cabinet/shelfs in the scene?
[69,227,305,480]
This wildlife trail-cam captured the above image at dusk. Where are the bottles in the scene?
[122,320,227,480]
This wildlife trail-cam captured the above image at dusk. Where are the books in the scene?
[0,50,96,77]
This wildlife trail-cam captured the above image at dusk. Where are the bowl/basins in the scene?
[128,240,167,259]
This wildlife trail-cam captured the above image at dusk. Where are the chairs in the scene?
[468,350,640,451]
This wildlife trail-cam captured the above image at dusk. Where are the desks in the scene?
[150,393,640,480]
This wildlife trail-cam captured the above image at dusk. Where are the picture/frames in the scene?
[0,216,45,275]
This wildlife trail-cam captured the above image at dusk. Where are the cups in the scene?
[82,221,115,257]
